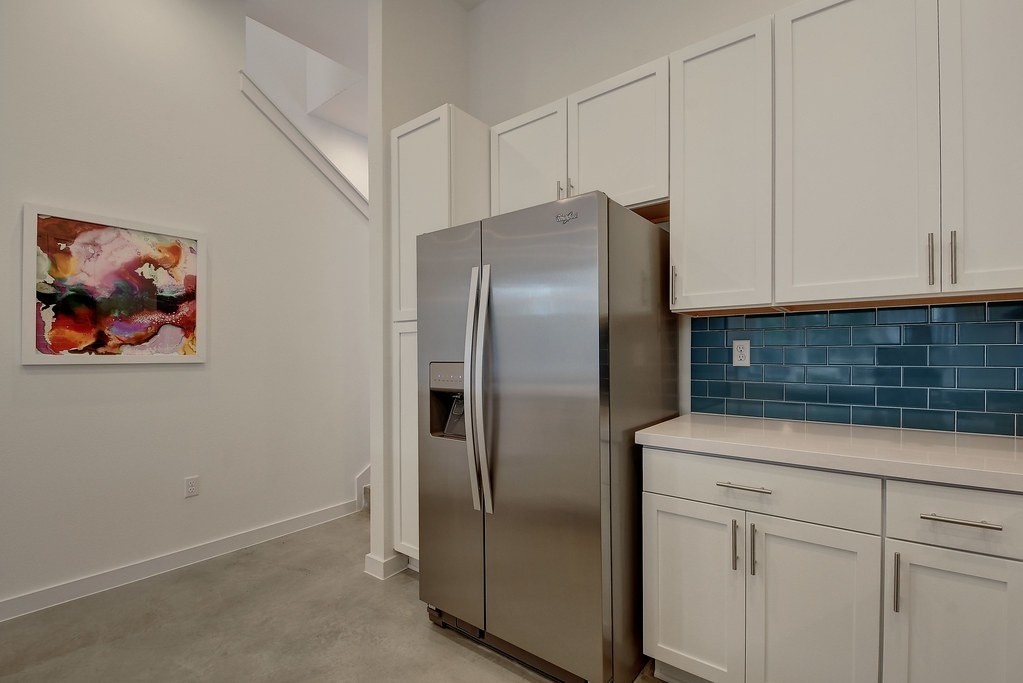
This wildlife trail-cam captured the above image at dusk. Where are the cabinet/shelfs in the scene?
[391,0,1023,683]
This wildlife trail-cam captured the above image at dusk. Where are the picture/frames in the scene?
[21,202,207,363]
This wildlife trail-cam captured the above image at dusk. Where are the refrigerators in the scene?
[416,190,680,683]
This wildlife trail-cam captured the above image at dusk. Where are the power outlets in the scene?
[184,475,199,499]
[732,340,751,367]
